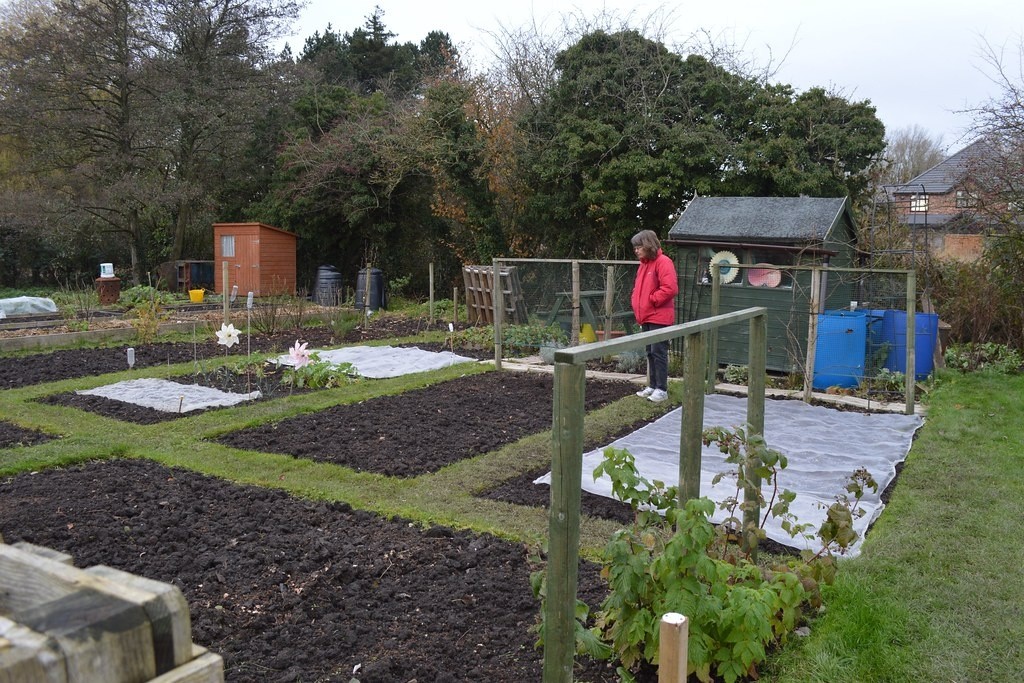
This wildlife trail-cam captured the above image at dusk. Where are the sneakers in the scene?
[636,386,654,397]
[647,388,668,402]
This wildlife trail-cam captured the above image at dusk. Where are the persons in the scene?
[631,230,679,403]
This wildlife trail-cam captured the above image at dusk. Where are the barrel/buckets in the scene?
[101,263,115,277]
[354,267,382,310]
[188,289,205,303]
[812,309,939,391]
[312,265,343,306]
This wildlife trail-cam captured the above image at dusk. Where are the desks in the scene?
[544,288,635,341]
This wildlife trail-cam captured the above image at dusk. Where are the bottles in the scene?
[127,348,134,367]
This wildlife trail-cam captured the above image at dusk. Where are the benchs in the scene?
[598,310,636,334]
[537,308,573,317]
